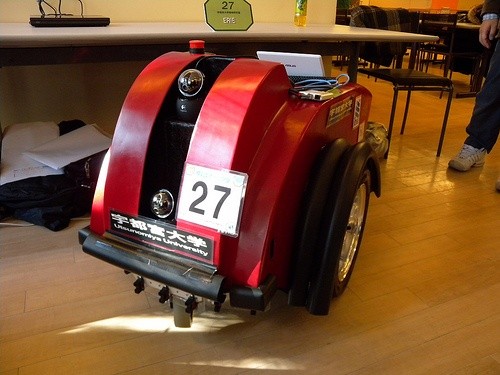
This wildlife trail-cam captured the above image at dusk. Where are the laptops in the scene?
[256,51,338,87]
[29,17,111,27]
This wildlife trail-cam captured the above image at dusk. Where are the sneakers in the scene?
[448,141,486,173]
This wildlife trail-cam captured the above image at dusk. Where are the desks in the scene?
[1,24,440,157]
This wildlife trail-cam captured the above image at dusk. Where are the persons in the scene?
[448,0,500,192]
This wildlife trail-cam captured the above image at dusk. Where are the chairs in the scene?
[358,12,459,156]
[336,5,497,94]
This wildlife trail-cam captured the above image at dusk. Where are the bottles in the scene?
[294,0,308,27]
